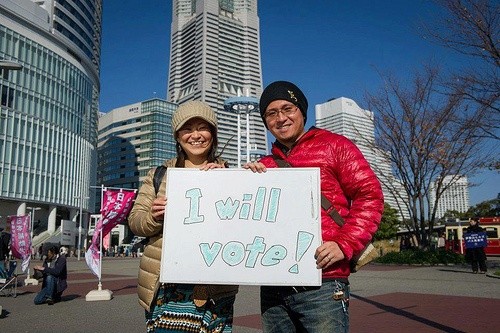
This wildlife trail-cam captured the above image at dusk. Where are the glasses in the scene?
[263,104,298,118]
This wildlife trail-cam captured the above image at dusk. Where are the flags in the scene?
[10,214,33,272]
[86,189,135,279]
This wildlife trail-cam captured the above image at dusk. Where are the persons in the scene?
[118,245,138,258]
[462,218,490,272]
[436,233,447,266]
[241,81,385,333]
[33,246,69,304]
[128,102,240,333]
[0,227,12,288]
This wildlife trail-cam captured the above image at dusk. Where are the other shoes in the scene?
[480,271,485,274]
[46,296,54,305]
[473,271,477,274]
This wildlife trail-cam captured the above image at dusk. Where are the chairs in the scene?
[0,259,18,298]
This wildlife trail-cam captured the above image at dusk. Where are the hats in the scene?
[259,80,308,130]
[171,101,218,135]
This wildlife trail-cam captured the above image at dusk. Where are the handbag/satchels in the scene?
[348,241,381,273]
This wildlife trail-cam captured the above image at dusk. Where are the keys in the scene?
[335,280,352,315]
